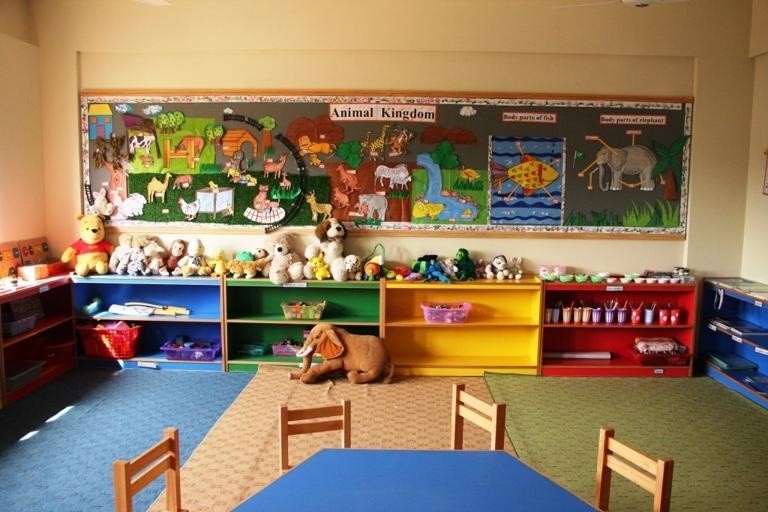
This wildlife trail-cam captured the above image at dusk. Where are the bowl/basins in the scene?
[560,274,691,284]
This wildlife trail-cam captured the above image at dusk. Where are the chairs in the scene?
[594,427,674,511]
[279,398,351,471]
[113,426,190,512]
[449,383,506,450]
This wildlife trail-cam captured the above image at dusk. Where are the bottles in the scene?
[673,265,689,276]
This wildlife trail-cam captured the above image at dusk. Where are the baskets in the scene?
[280,300,326,319]
[160,335,221,360]
[272,346,303,355]
[420,301,471,322]
[76,324,142,359]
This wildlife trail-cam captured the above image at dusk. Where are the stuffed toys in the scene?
[61,211,116,276]
[303,217,477,284]
[61,211,526,285]
[108,233,274,279]
[264,231,306,284]
[288,323,398,386]
[477,254,523,281]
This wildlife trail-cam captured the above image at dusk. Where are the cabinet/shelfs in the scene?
[543,284,693,371]
[224,278,381,369]
[0,276,70,410]
[384,284,540,373]
[73,278,224,368]
[698,277,767,405]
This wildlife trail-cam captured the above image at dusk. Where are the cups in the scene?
[544,307,680,326]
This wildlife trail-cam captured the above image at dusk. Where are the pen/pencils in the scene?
[550,298,657,310]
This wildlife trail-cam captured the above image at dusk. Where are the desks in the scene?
[231,448,594,512]
